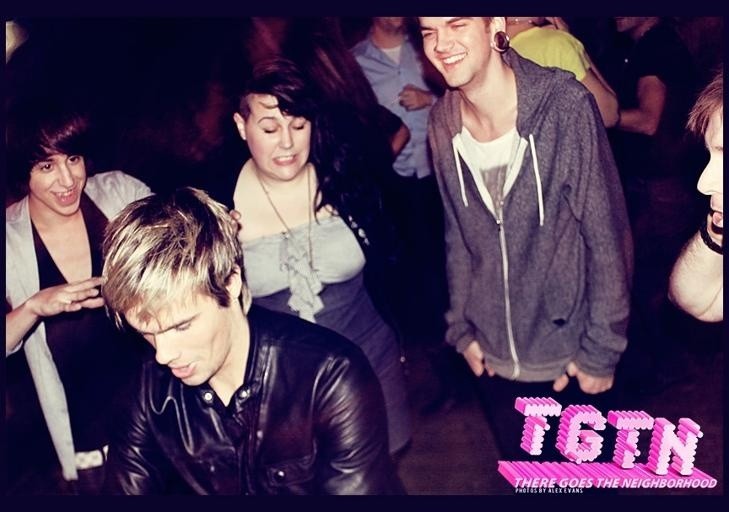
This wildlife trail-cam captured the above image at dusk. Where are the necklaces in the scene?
[254,165,314,270]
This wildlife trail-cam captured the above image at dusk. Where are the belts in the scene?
[74,446,109,469]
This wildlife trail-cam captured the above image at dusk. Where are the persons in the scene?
[100,187,409,495]
[292,14,723,461]
[219,57,415,452]
[5,105,159,495]
